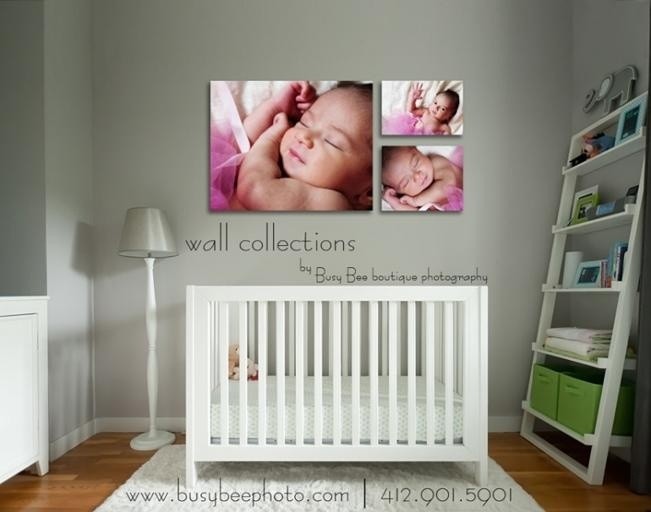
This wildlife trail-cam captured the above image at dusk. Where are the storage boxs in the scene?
[530,362,635,439]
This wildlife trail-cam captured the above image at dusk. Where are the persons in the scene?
[229,81,373,211]
[382,146,463,211]
[407,82,459,134]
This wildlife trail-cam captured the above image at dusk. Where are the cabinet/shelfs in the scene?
[519,91,648,488]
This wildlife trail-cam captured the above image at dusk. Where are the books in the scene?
[609,242,628,281]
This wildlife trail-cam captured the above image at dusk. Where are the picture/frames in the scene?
[573,259,609,289]
[571,184,599,224]
[615,91,648,147]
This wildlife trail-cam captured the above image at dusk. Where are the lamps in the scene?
[117,206,179,452]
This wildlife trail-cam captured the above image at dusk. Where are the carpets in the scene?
[87,442,546,511]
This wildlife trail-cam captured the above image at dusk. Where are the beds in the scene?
[186,285,490,487]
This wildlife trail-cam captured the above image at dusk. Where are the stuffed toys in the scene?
[229,344,239,376]
[234,359,257,379]
